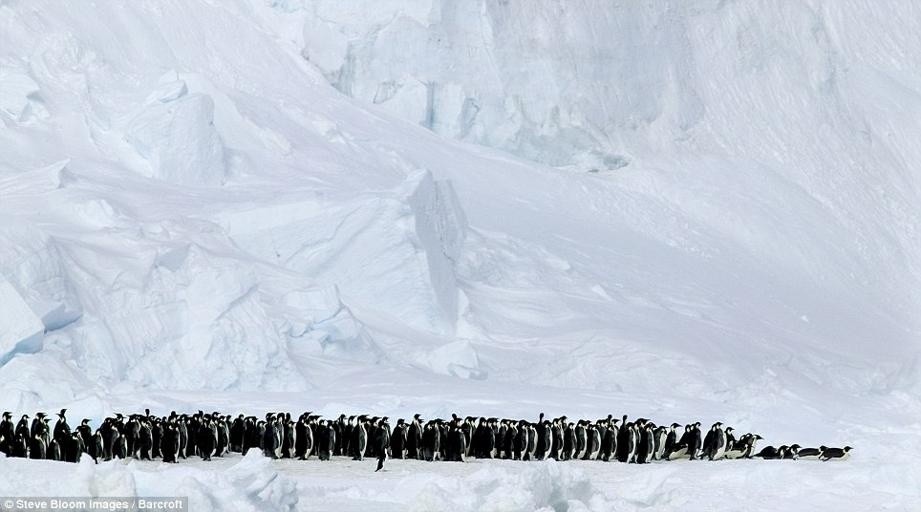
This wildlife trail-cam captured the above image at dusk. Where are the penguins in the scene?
[374,444,390,472]
[0,408,855,463]
